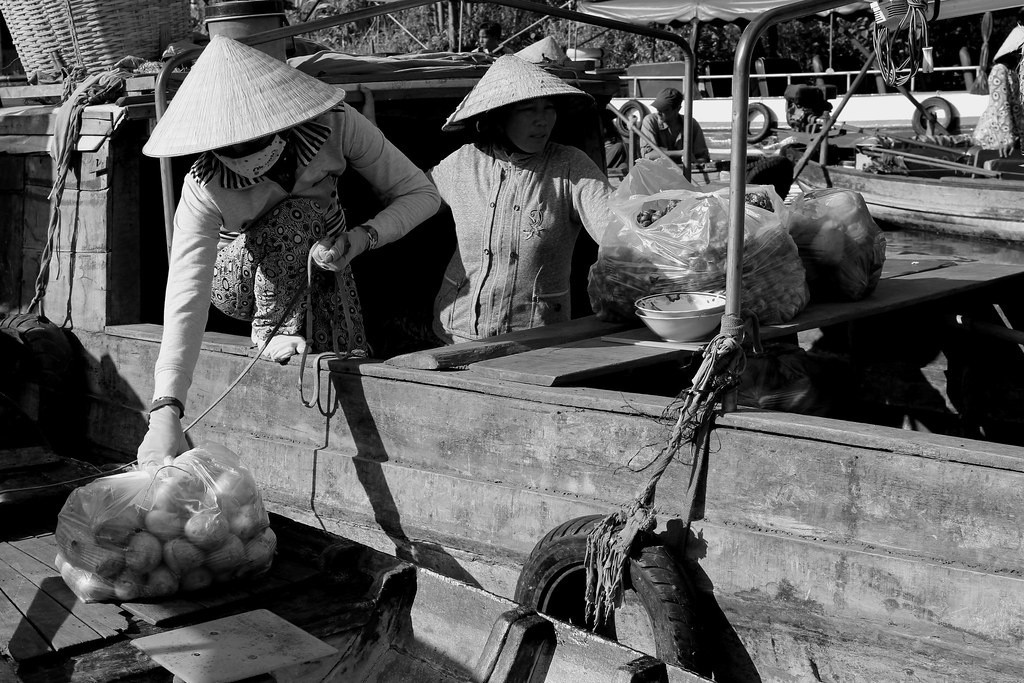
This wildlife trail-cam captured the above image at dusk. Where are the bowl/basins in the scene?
[634,292,727,342]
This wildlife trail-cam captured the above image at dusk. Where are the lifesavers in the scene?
[912,97,953,136]
[616,100,645,138]
[746,103,772,144]
[513,515,713,680]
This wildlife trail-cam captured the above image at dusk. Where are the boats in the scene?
[0,0,1023,683]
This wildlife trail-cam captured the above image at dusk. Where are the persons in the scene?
[359,54,617,346]
[471,21,514,56]
[640,88,711,163]
[137,34,442,467]
[971,27,1024,157]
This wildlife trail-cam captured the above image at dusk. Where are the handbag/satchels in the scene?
[587,155,887,320]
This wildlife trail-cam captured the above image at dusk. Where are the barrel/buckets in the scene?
[203,0,289,64]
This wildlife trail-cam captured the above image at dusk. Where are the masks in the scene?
[210,136,287,179]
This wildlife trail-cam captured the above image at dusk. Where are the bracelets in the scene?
[149,398,184,419]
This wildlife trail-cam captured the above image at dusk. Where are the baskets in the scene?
[0,0,192,82]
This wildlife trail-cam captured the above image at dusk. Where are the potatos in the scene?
[787,191,887,301]
[53,449,274,603]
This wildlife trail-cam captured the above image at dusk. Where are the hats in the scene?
[651,87,683,111]
[513,36,567,65]
[142,34,346,158]
[440,55,595,132]
[993,25,1024,61]
